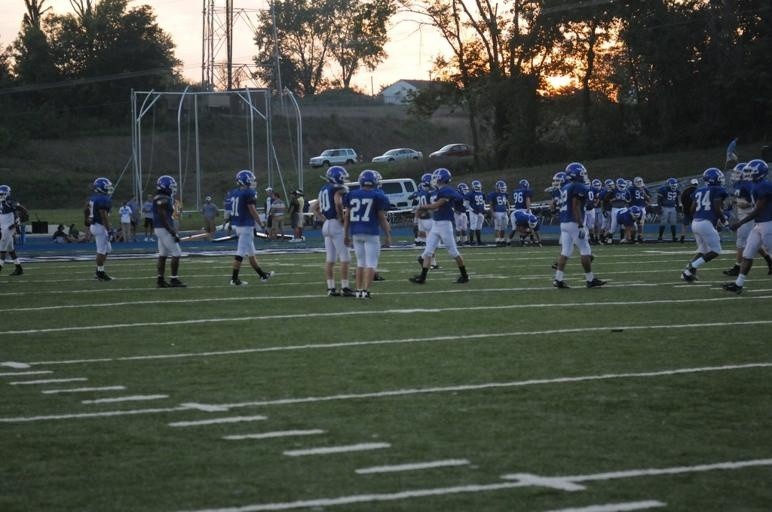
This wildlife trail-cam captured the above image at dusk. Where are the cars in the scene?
[371,147,424,164]
[428,143,473,159]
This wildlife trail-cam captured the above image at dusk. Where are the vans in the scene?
[342,177,418,212]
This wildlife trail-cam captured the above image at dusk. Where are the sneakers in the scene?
[288,238,302,243]
[414,237,426,246]
[409,255,469,283]
[168,278,187,287]
[9,265,23,275]
[157,275,169,287]
[260,271,275,282]
[656,235,685,243]
[681,265,772,295]
[590,234,644,244]
[97,271,112,280]
[553,279,569,288]
[587,278,607,288]
[230,280,248,286]
[456,238,543,247]
[327,272,385,299]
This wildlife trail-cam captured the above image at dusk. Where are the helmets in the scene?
[630,206,640,220]
[236,170,257,189]
[528,215,538,229]
[551,162,588,188]
[667,178,678,191]
[326,166,383,188]
[93,178,114,194]
[0,185,11,201]
[417,168,452,192]
[156,175,177,195]
[456,179,530,194]
[586,177,643,191]
[703,159,769,186]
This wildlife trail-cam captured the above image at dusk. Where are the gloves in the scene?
[578,224,586,239]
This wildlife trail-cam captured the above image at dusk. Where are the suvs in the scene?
[308,148,359,169]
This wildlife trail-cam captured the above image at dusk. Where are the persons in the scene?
[7,200,29,246]
[407,168,544,284]
[51,194,182,244]
[720,160,772,295]
[552,162,607,288]
[723,163,772,276]
[586,177,738,246]
[318,166,355,297]
[263,186,307,243]
[230,170,274,286]
[723,137,739,167]
[371,170,385,282]
[544,172,594,269]
[0,184,24,276]
[680,167,728,283]
[153,176,188,288]
[89,178,113,281]
[201,190,233,240]
[342,170,390,300]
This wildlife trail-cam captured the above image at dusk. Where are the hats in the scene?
[691,179,698,184]
